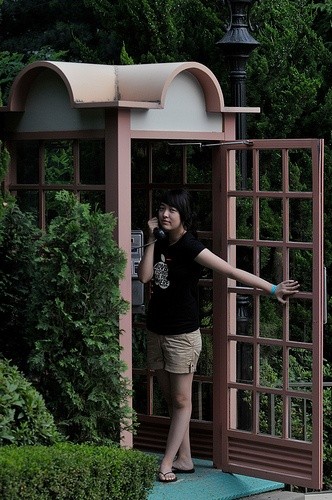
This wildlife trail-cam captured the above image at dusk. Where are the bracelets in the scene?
[270,284,277,296]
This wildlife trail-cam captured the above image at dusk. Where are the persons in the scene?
[137,188,301,482]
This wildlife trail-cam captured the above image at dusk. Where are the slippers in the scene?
[172,465,195,473]
[158,471,178,482]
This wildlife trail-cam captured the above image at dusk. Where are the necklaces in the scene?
[170,229,187,245]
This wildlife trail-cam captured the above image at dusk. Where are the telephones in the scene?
[152,204,167,238]
